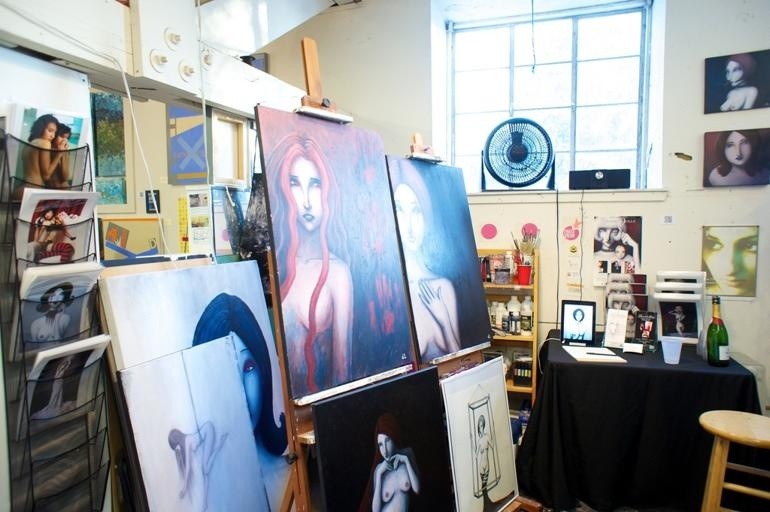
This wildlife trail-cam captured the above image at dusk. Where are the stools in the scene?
[696,408,770,512]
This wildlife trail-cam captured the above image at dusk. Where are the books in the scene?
[562,344,626,365]
[99,213,163,258]
[167,102,207,184]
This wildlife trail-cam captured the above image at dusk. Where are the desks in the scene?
[529,331,756,511]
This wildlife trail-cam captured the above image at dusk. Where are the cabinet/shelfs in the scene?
[476,244,540,448]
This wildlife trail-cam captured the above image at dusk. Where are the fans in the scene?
[480,116,558,191]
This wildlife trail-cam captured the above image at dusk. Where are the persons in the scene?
[476,413,495,488]
[570,308,589,340]
[388,157,459,363]
[703,226,758,296]
[669,304,685,334]
[267,134,354,393]
[13,111,83,421]
[593,218,639,337]
[169,420,231,510]
[192,293,295,511]
[360,414,421,511]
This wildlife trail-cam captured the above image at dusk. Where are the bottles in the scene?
[489,254,515,283]
[708,295,731,366]
[488,294,533,333]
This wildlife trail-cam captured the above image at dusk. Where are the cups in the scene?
[662,336,684,365]
[517,264,531,283]
